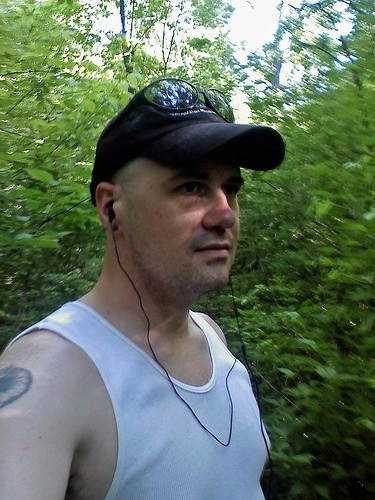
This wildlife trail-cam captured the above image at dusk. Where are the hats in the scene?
[90,99,283,206]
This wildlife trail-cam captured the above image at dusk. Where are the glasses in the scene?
[96,78,233,143]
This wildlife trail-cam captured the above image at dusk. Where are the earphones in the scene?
[108,200,118,231]
